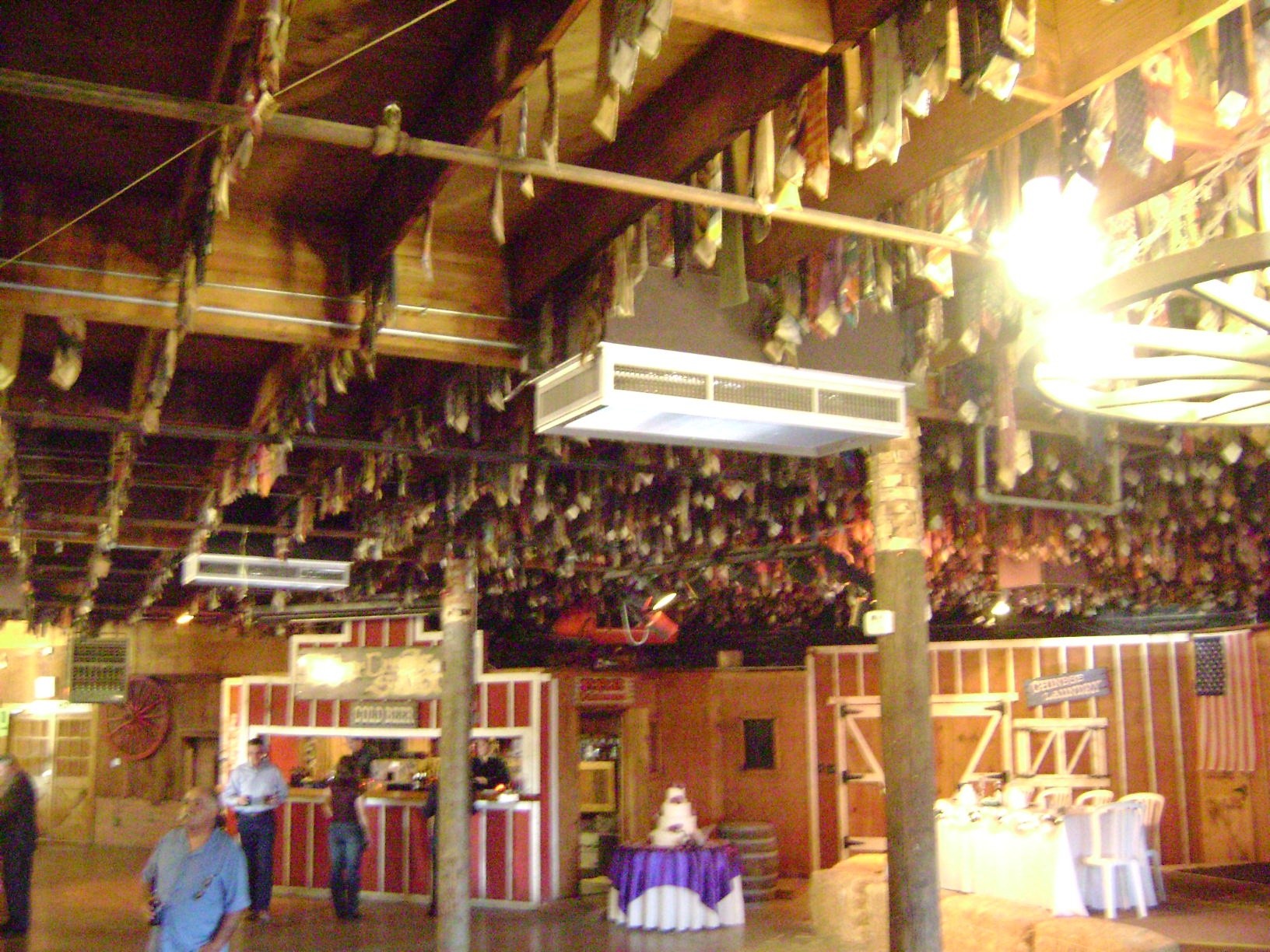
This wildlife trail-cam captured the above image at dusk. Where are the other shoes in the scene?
[246,910,269,920]
[427,909,438,916]
[336,909,363,920]
[0,924,27,939]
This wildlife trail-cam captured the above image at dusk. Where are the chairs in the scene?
[934,781,1166,920]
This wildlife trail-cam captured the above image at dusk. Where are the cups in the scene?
[150,899,160,925]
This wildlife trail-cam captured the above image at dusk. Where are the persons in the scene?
[139,786,252,952]
[321,755,370,924]
[290,737,518,798]
[219,738,288,924]
[422,762,480,915]
[0,753,37,938]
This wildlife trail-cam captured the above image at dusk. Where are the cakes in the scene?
[649,781,706,847]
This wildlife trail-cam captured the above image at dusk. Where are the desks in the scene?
[605,838,747,931]
[930,796,1160,918]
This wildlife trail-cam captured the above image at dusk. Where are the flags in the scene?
[1194,630,1258,773]
